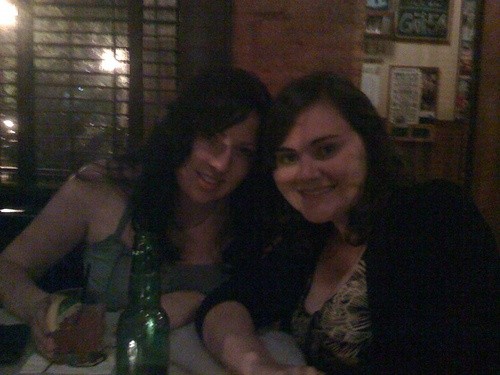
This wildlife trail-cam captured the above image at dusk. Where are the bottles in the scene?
[115,232,171,375]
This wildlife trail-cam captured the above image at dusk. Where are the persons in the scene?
[195,70,500,375]
[0,69,274,372]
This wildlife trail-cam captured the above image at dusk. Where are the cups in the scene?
[53,289,108,367]
